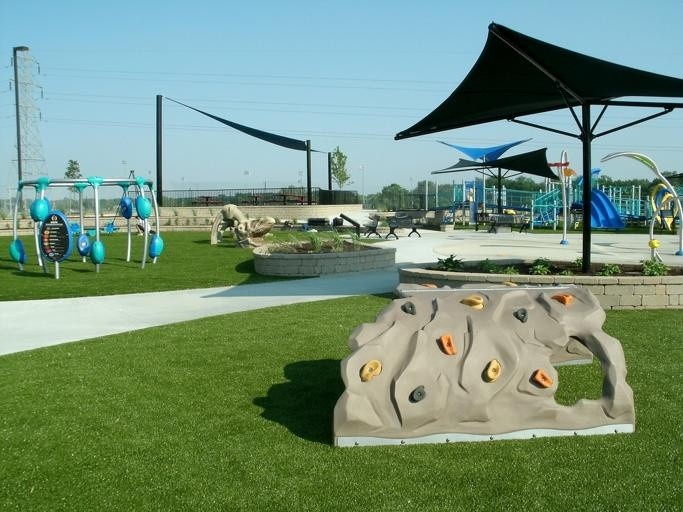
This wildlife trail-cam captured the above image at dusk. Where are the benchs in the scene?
[363,214,423,239]
